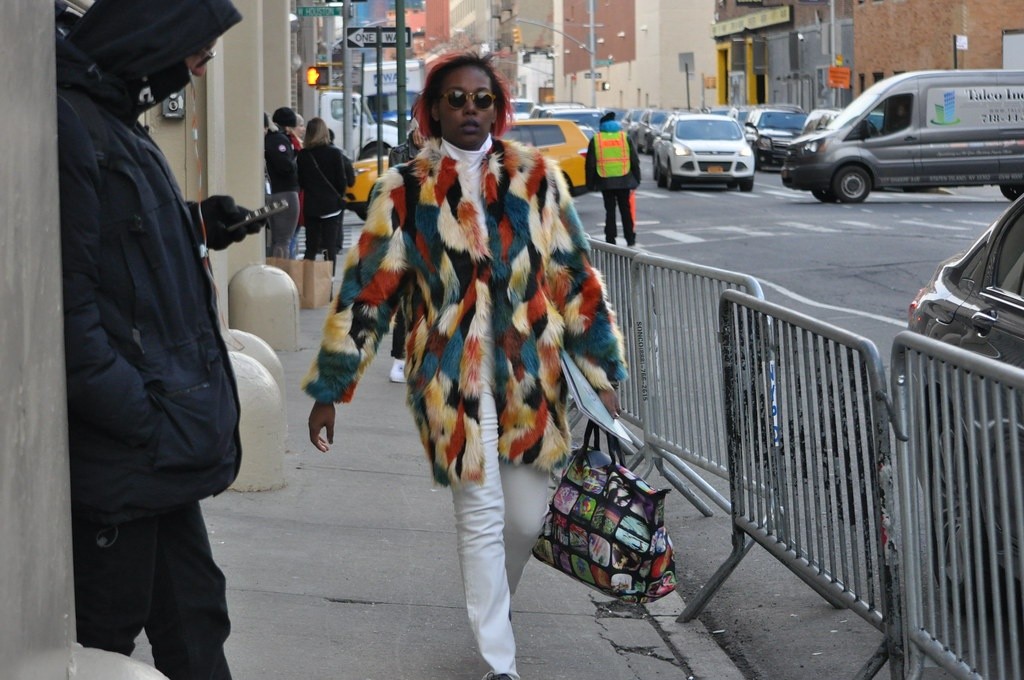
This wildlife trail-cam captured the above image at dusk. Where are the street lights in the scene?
[512,16,597,108]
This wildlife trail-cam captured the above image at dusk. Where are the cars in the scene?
[505,97,886,176]
[906,192,1024,370]
[652,110,760,193]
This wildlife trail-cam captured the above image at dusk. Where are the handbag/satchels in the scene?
[302,249,333,309]
[265,246,303,308]
[531,418,677,601]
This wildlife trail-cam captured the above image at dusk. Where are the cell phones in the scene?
[226,200,288,232]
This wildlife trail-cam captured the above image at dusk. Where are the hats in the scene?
[600,112,615,124]
[272,107,297,127]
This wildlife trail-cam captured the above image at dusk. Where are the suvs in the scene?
[341,118,590,233]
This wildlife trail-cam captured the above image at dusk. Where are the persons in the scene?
[878,103,910,136]
[388,106,429,383]
[303,49,631,679]
[264,106,356,302]
[57,1,268,680]
[586,113,641,246]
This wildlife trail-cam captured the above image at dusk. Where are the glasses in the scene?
[195,47,216,69]
[432,88,497,111]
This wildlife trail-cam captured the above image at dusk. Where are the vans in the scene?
[780,68,1024,203]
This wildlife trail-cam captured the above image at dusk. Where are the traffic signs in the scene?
[347,24,413,49]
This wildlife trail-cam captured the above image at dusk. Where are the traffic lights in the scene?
[511,25,522,45]
[305,64,330,87]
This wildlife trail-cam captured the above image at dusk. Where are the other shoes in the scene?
[389,359,407,383]
[626,238,635,246]
[605,237,616,245]
[487,673,511,680]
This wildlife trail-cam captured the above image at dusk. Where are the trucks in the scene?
[314,57,428,166]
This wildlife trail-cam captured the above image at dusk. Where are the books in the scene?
[558,349,633,444]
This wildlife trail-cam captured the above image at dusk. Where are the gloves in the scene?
[190,196,271,250]
